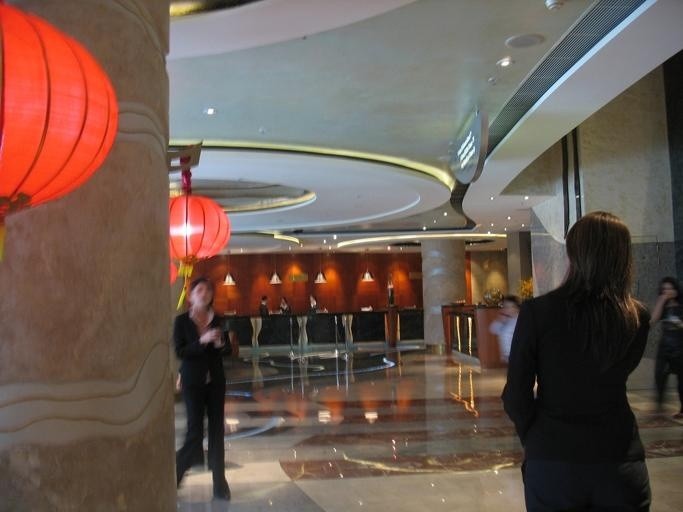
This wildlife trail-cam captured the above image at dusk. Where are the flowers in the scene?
[517,278,534,301]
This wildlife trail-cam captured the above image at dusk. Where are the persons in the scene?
[501,211,651,512]
[490,296,521,362]
[648,276,682,419]
[173,278,231,501]
[259,295,270,316]
[279,296,291,315]
[307,294,321,314]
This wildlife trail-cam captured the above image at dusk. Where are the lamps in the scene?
[268,253,282,285]
[221,250,235,287]
[311,248,329,284]
[360,247,374,282]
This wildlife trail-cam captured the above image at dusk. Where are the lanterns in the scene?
[0,0,118,262]
[169,196,231,310]
[169,260,178,288]
